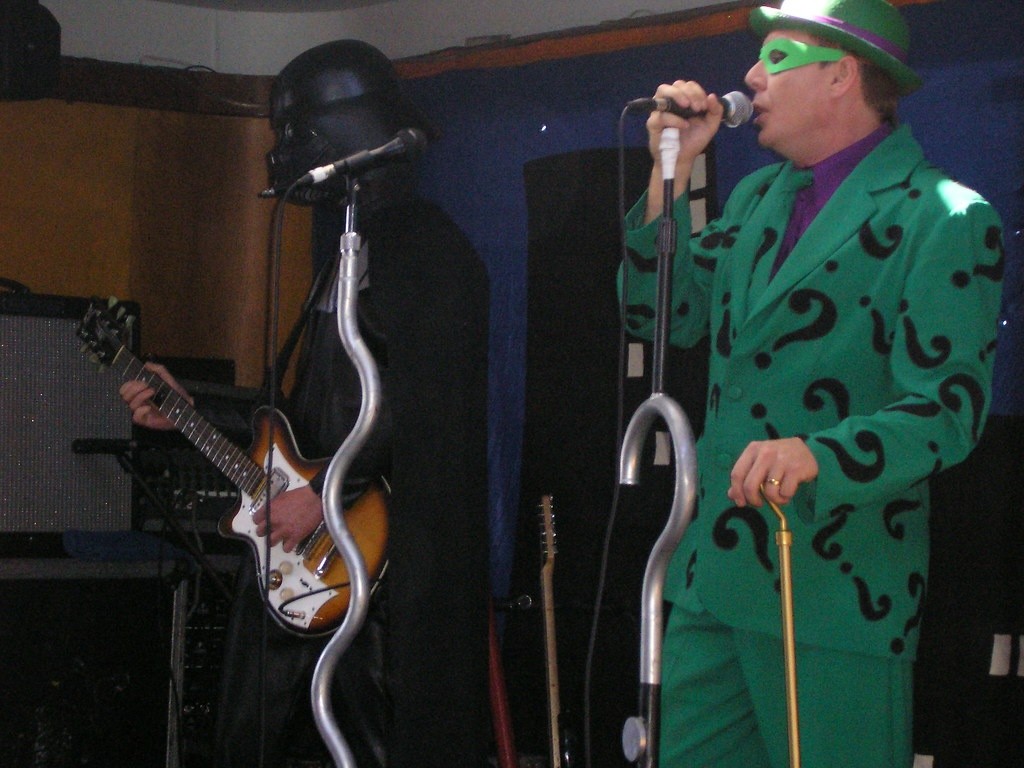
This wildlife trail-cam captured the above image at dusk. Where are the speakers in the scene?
[0,292,143,558]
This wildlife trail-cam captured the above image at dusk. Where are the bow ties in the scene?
[788,171,817,188]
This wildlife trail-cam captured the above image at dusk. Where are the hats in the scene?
[745,2,930,92]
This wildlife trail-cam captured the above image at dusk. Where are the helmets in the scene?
[266,40,452,145]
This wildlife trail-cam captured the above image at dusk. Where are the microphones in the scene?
[627,91,754,128]
[293,127,427,188]
[72,438,138,455]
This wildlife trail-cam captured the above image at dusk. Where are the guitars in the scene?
[73,294,401,640]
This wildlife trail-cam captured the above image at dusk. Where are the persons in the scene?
[118,40,496,768]
[617,0,1006,768]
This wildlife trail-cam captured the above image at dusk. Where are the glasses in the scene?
[756,41,844,77]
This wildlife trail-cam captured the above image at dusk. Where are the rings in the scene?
[764,478,781,485]
